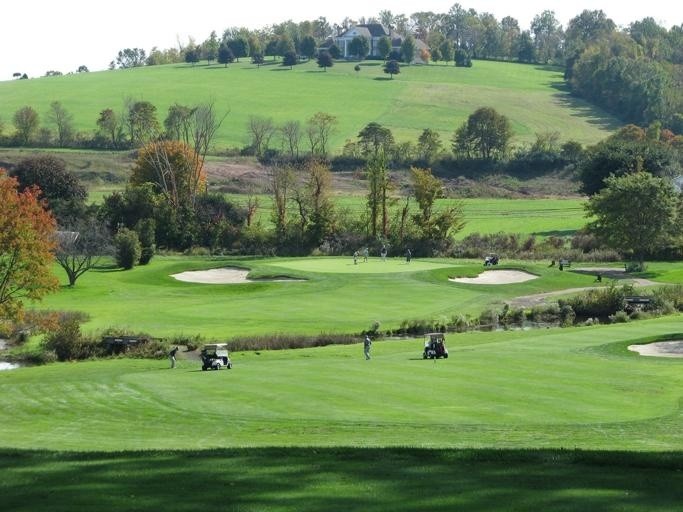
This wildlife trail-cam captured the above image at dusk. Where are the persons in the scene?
[353,250,359,264]
[170,347,178,368]
[381,244,387,262]
[363,247,369,262]
[490,255,499,265]
[364,335,372,360]
[407,249,411,263]
